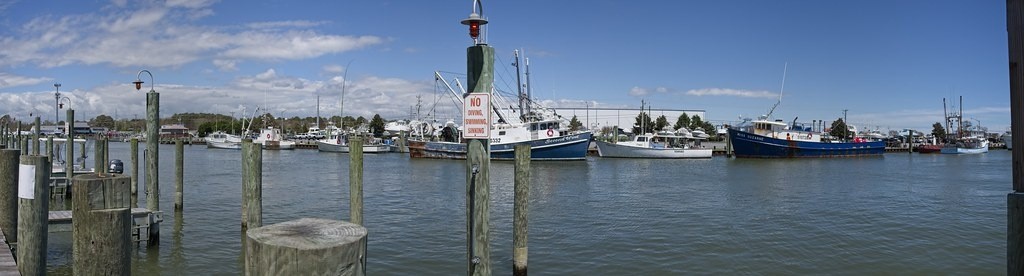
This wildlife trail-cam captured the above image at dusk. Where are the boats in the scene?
[408,49,592,162]
[727,115,886,158]
[204,131,243,149]
[315,131,391,154]
[251,126,297,150]
[1004,125,1012,150]
[940,141,990,154]
[916,144,945,153]
[595,133,713,159]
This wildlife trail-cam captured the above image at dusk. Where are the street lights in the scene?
[58,97,74,198]
[132,69,160,244]
[29,108,40,155]
[13,113,21,150]
[460,0,495,276]
